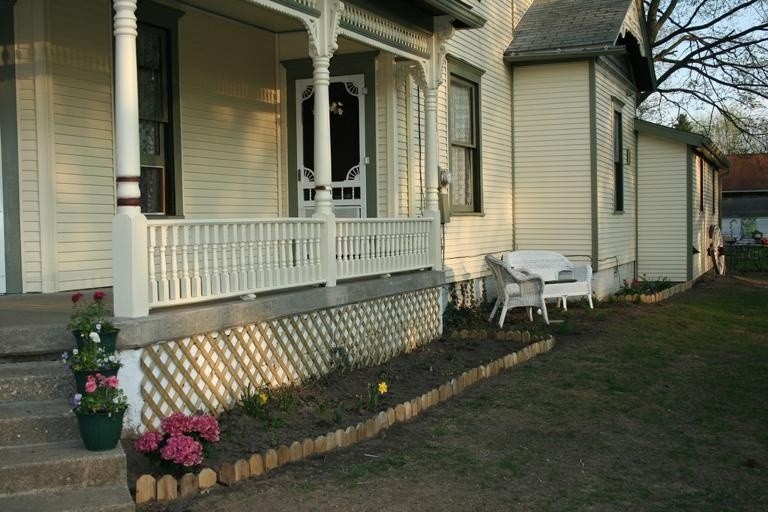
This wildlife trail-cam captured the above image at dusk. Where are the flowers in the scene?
[135,413,221,468]
[58,287,131,422]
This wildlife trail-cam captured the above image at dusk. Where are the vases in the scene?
[75,410,124,452]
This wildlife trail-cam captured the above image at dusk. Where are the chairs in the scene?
[483,249,596,328]
